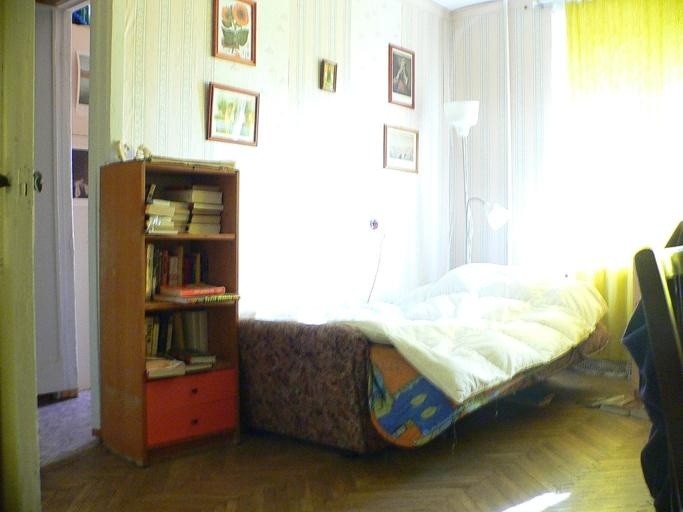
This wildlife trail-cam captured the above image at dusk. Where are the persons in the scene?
[393,57,411,96]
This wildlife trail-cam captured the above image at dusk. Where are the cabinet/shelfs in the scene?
[97,157,243,468]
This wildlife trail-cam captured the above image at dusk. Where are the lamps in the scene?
[444,99,511,265]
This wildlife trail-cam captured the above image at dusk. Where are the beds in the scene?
[239,261,609,460]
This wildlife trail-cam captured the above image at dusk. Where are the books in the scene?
[144,183,240,379]
[507,392,555,409]
[575,388,651,421]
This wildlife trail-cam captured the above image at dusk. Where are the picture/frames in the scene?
[205,82,261,147]
[321,58,338,93]
[388,43,416,110]
[213,0,258,67]
[383,123,419,174]
